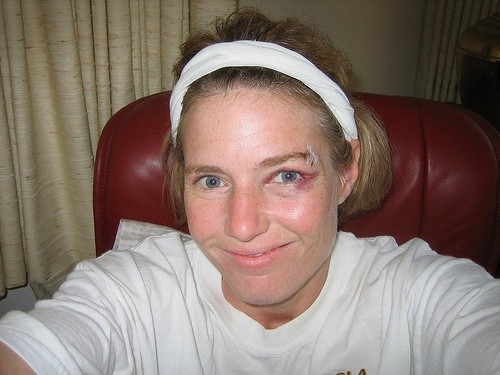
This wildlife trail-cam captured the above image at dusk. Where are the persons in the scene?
[0,8,500,375]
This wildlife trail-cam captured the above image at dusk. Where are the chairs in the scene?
[92,90,500,278]
